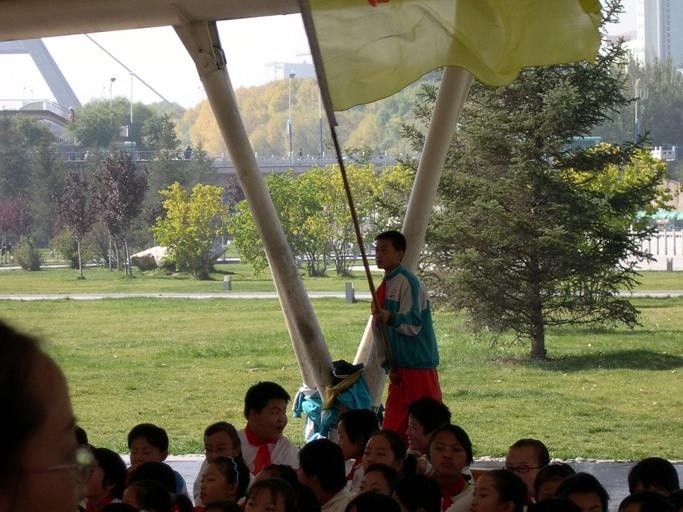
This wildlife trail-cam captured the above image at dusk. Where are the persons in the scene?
[75,379,683,510]
[366,230,445,436]
[0,317,83,512]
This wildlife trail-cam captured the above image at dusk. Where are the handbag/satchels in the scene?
[332,360,364,386]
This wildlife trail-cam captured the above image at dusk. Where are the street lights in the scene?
[633,77,640,143]
[107,76,117,127]
[288,72,296,156]
[128,72,135,123]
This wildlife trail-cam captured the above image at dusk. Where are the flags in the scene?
[296,1,604,127]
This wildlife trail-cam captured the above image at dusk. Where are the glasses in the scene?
[502,464,539,474]
[28,442,98,486]
